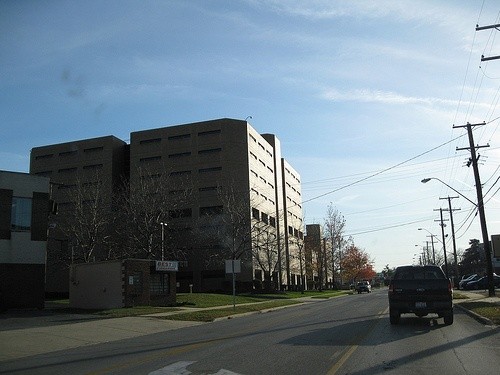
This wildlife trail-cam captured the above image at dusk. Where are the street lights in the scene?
[412,244,432,266]
[420,176,496,297]
[418,227,449,278]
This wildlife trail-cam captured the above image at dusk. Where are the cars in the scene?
[459,273,479,289]
[467,273,500,290]
[357,281,372,294]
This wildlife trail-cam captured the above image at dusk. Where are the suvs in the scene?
[388,263,453,327]
